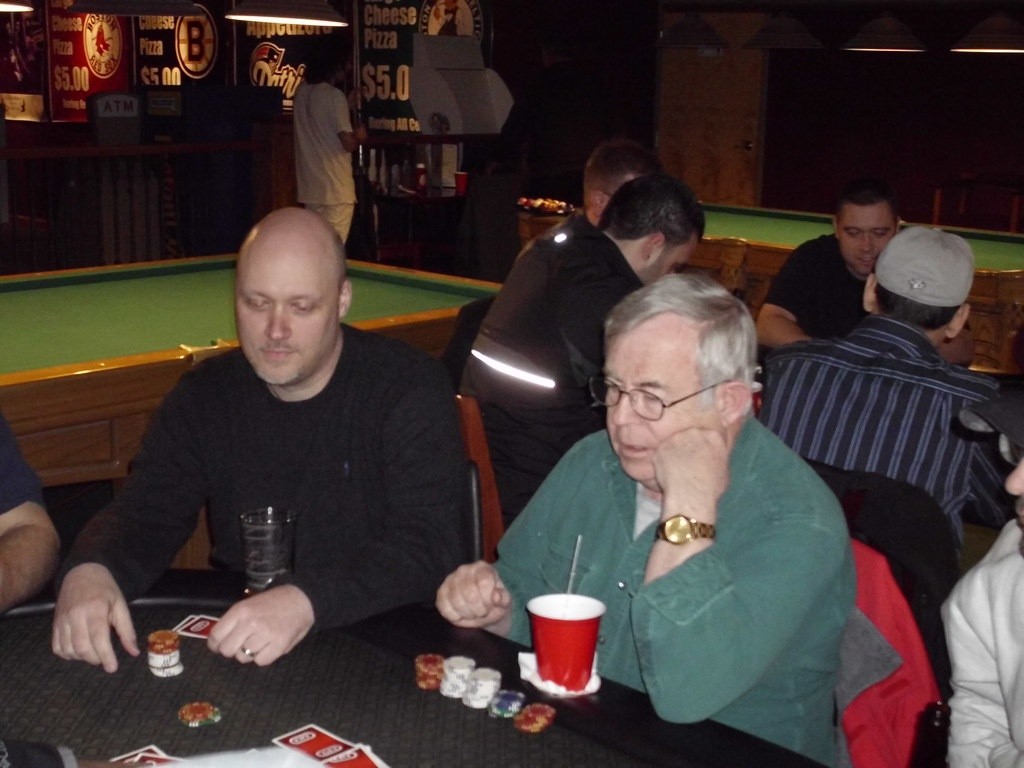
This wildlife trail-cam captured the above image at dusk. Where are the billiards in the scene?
[517,196,575,212]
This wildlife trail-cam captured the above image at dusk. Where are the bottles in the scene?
[367,149,427,199]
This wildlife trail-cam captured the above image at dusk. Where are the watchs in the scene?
[654,515,716,545]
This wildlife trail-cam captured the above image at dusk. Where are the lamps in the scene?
[68,0,204,16]
[225,1,350,27]
[840,1,927,52]
[0,0,34,12]
[951,0,1024,52]
[742,1,824,49]
[652,1,729,48]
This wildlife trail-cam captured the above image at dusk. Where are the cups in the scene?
[240,507,291,596]
[527,594,606,691]
[454,173,468,196]
[751,382,763,417]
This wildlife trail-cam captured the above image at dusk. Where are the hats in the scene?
[958,377,1024,447]
[874,226,974,307]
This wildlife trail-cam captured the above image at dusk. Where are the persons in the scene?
[757,176,973,367]
[514,136,665,265]
[52,209,473,664]
[753,225,1018,768]
[292,40,367,247]
[458,168,704,532]
[435,268,855,768]
[0,410,61,623]
[940,385,1024,768]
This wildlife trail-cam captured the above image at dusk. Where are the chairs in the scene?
[456,394,506,564]
[807,453,962,700]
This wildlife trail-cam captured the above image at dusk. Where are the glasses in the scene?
[588,377,733,420]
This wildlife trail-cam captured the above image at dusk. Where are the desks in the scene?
[518,198,1023,381]
[371,188,478,270]
[0,570,831,768]
[0,251,508,570]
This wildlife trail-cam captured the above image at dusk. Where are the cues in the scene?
[355,51,366,251]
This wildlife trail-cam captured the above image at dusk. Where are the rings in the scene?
[242,646,256,658]
